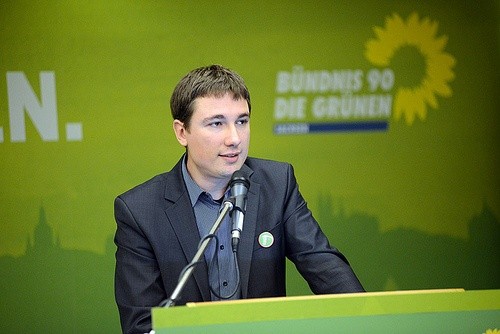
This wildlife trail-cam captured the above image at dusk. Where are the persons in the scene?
[112,62,366,334]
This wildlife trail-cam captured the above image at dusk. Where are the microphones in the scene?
[230,171,250,252]
